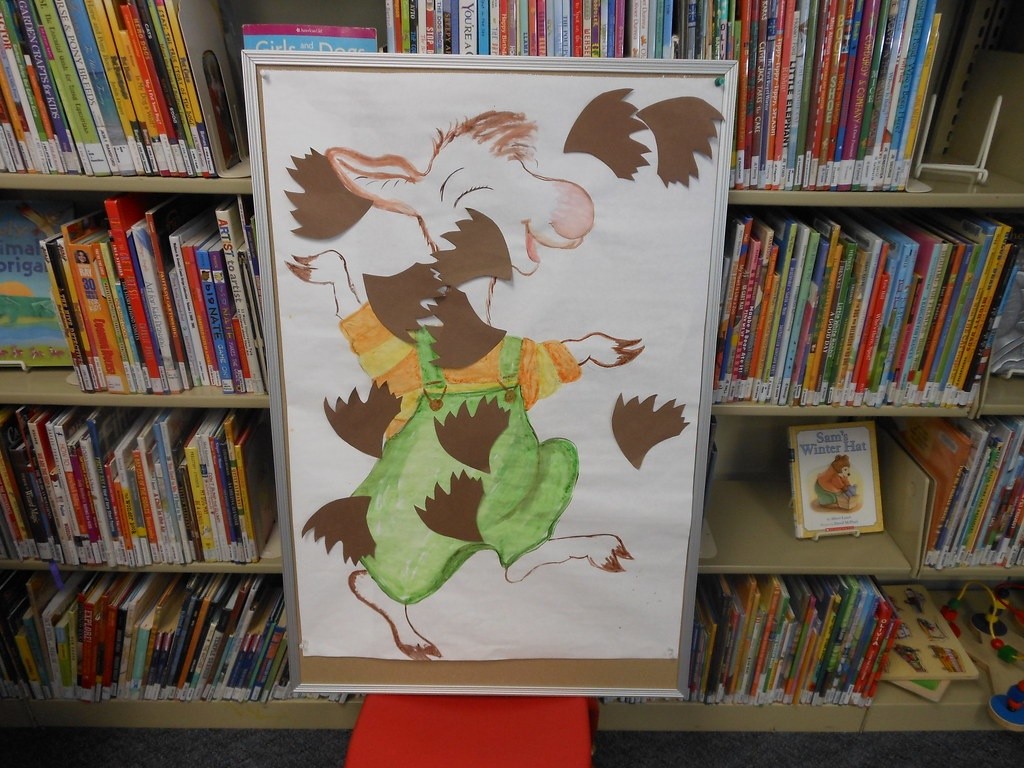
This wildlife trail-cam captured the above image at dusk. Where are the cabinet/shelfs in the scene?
[0,0,1024,733]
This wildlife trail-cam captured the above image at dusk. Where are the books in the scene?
[0,0,949,190]
[701,416,1024,571]
[1,569,367,704]
[0,195,269,394]
[1,404,278,569]
[712,203,1024,407]
[600,571,986,709]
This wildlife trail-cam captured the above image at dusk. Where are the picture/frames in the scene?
[235,47,742,695]
